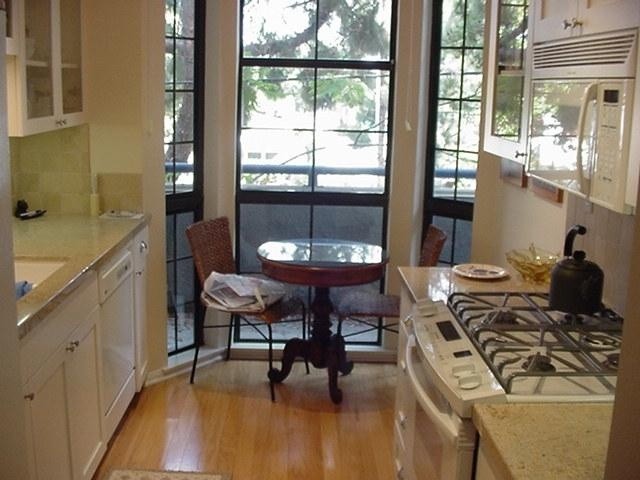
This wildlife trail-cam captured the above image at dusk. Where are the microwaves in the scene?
[525,78,638,216]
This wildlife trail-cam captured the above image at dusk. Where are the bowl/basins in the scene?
[505,249,563,284]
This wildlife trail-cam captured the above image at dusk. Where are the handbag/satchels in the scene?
[201,271,286,314]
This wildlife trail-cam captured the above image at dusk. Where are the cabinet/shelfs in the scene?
[0,218,151,478]
[483,1,639,165]
[6,1,92,138]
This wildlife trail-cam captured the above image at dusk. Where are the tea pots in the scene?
[550,224,603,313]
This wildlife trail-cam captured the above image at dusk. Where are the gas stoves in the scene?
[408,290,624,418]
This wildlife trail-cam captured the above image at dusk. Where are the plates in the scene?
[452,263,507,281]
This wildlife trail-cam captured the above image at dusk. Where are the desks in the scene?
[257,236,392,404]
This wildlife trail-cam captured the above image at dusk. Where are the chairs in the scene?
[337,224,447,344]
[182,218,310,404]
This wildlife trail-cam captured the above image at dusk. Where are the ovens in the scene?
[404,334,476,480]
